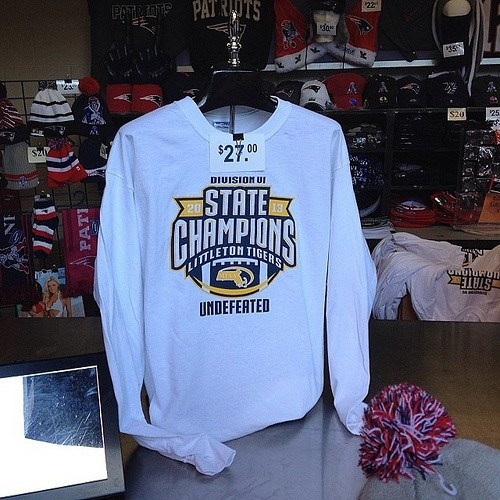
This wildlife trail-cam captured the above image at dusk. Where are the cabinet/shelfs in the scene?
[315,106,500,240]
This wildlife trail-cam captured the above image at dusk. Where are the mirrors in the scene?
[0,352,124,500]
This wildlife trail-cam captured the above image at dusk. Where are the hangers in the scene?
[192,24,277,114]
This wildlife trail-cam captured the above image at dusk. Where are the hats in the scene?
[0,1,500,226]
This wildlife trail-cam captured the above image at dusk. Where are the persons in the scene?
[22,276,72,317]
